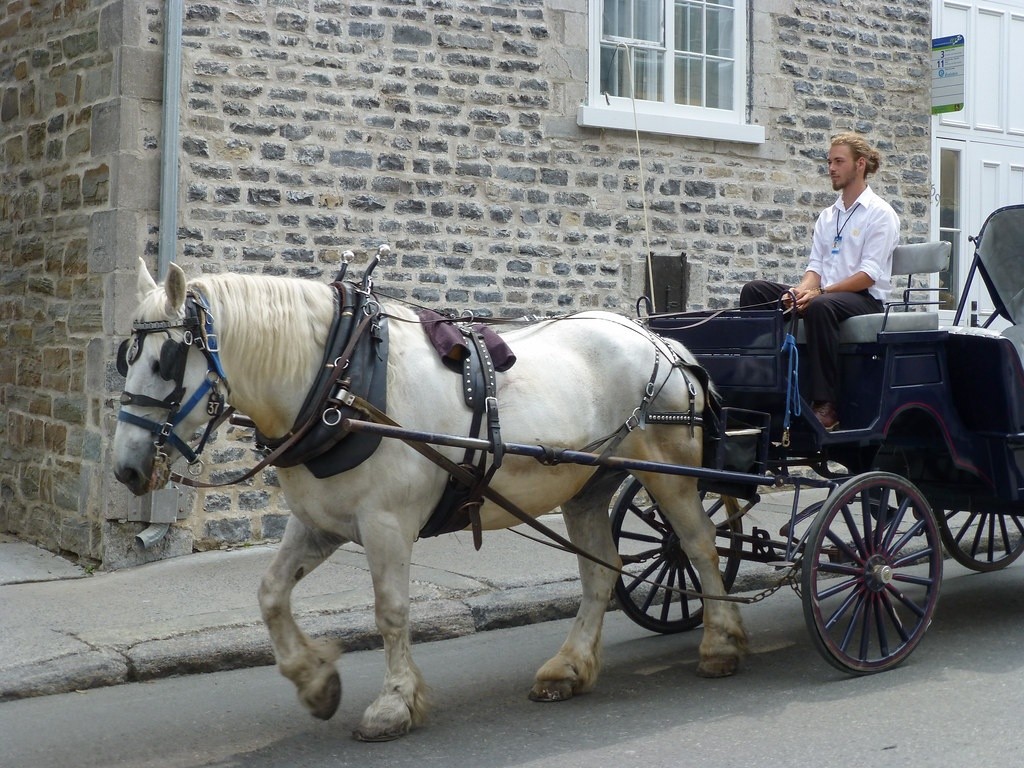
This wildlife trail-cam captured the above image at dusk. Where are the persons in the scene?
[740,134,901,431]
[833,242,837,248]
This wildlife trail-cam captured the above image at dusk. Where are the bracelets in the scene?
[817,287,826,294]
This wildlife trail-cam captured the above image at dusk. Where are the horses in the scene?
[102,252,757,746]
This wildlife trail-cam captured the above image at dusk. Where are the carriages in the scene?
[111,204,1024,743]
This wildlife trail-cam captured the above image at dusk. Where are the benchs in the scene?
[782,240,952,344]
[938,323,1024,435]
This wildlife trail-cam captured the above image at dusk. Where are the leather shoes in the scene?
[812,400,841,432]
